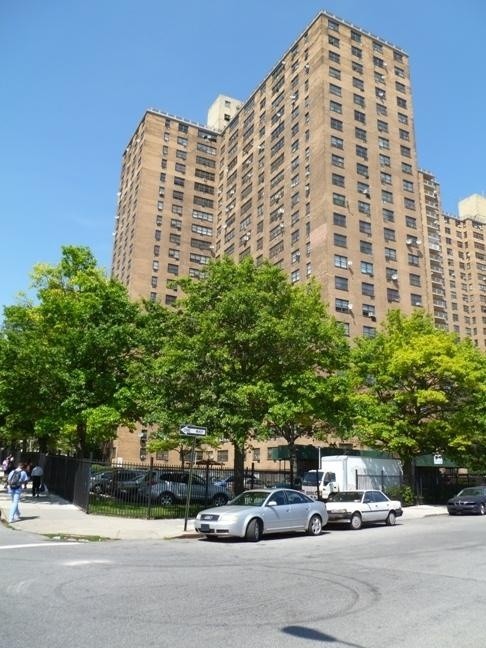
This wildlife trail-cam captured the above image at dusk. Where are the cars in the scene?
[446,486,486,515]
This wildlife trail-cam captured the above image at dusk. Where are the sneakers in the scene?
[9,513,21,523]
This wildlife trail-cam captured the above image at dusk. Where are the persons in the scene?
[3,453,44,498]
[7,463,28,523]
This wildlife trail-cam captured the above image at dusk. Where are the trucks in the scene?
[302,455,403,500]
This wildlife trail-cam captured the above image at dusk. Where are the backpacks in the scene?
[10,469,23,489]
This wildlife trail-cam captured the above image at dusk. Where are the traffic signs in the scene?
[179,424,207,437]
